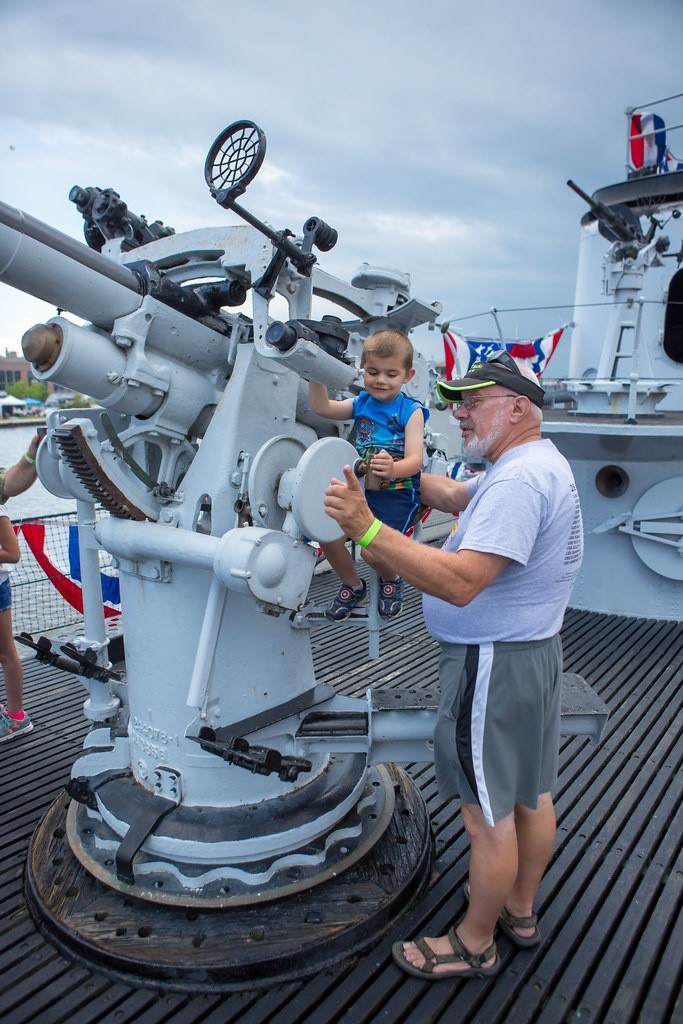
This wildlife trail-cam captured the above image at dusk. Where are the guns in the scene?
[566,177,658,244]
[0,113,453,447]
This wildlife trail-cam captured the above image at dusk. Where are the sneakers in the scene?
[324,578,368,623]
[0,704,33,742]
[378,575,404,617]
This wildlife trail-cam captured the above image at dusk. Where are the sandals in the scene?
[391,924,500,978]
[464,879,542,946]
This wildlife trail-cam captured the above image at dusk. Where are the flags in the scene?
[442,328,563,381]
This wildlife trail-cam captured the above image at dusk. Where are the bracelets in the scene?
[358,518,383,548]
[24,452,36,465]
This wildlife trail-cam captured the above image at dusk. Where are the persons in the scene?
[0,434,41,742]
[324,360,587,980]
[308,329,429,623]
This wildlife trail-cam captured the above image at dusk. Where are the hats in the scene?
[437,362,545,408]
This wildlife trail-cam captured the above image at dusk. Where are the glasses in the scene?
[487,349,521,375]
[459,395,516,410]
